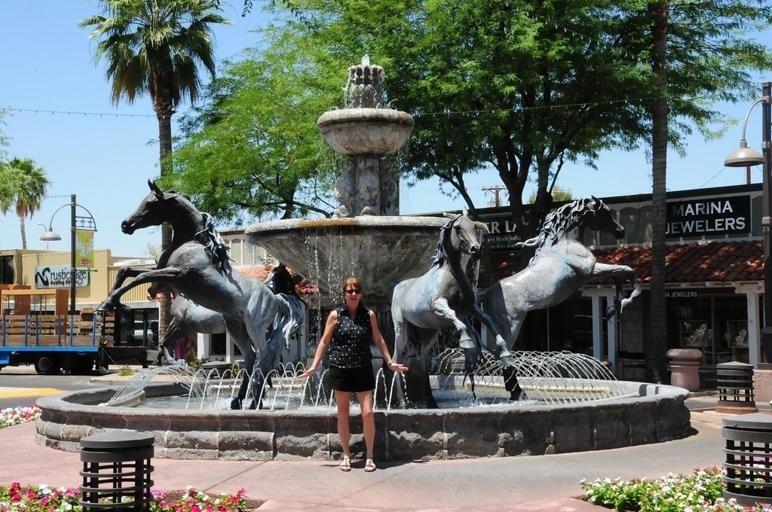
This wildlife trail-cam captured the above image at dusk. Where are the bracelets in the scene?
[387,361,394,367]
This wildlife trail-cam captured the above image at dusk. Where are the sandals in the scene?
[339,454,351,471]
[364,457,376,472]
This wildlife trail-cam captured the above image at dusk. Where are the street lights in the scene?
[39,193,98,311]
[723,80,772,370]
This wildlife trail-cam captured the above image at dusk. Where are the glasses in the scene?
[345,288,362,294]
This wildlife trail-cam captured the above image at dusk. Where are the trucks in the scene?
[1,314,103,374]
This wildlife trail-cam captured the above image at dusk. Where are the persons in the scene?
[262,259,292,295]
[298,276,409,472]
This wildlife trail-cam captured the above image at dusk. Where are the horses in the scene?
[94,178,303,411]
[149,280,227,367]
[387,205,513,359]
[483,195,643,402]
